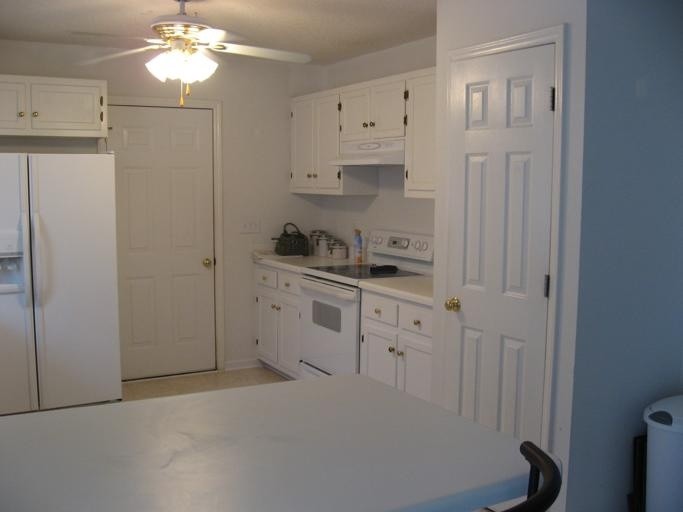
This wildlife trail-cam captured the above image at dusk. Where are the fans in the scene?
[63,0,314,68]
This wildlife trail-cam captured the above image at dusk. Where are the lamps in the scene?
[144,39,221,107]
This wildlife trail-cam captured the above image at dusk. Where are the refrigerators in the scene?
[0,151,123,417]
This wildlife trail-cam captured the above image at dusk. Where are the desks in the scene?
[0,371,565,512]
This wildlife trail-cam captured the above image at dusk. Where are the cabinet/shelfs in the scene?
[359,288,432,402]
[290,66,436,199]
[254,262,301,381]
[0,75,108,140]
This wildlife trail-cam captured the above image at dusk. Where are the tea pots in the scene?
[271,221,307,256]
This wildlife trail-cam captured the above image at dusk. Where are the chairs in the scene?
[484,441,562,512]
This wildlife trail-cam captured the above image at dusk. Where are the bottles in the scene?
[309,230,347,260]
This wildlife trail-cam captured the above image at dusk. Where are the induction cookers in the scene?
[302,260,426,285]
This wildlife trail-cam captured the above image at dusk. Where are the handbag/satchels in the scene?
[275,223,308,255]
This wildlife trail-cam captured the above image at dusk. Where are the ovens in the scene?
[298,275,361,380]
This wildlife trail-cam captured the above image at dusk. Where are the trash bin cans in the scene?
[643,395,683,511]
